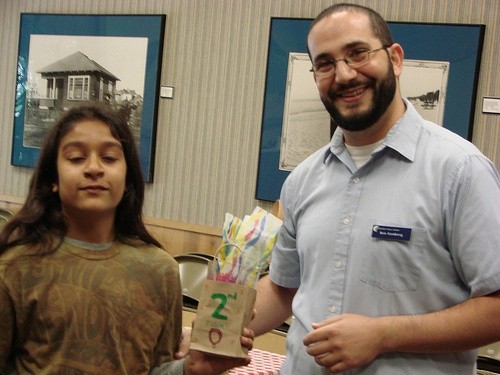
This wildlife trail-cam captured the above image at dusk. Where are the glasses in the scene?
[310,43,391,75]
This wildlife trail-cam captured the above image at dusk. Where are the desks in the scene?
[181,323,286,374]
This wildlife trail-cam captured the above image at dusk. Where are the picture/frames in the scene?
[254,17,486,203]
[10,11,167,185]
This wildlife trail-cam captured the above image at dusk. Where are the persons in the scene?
[0,103,256,375]
[174,2,500,375]
